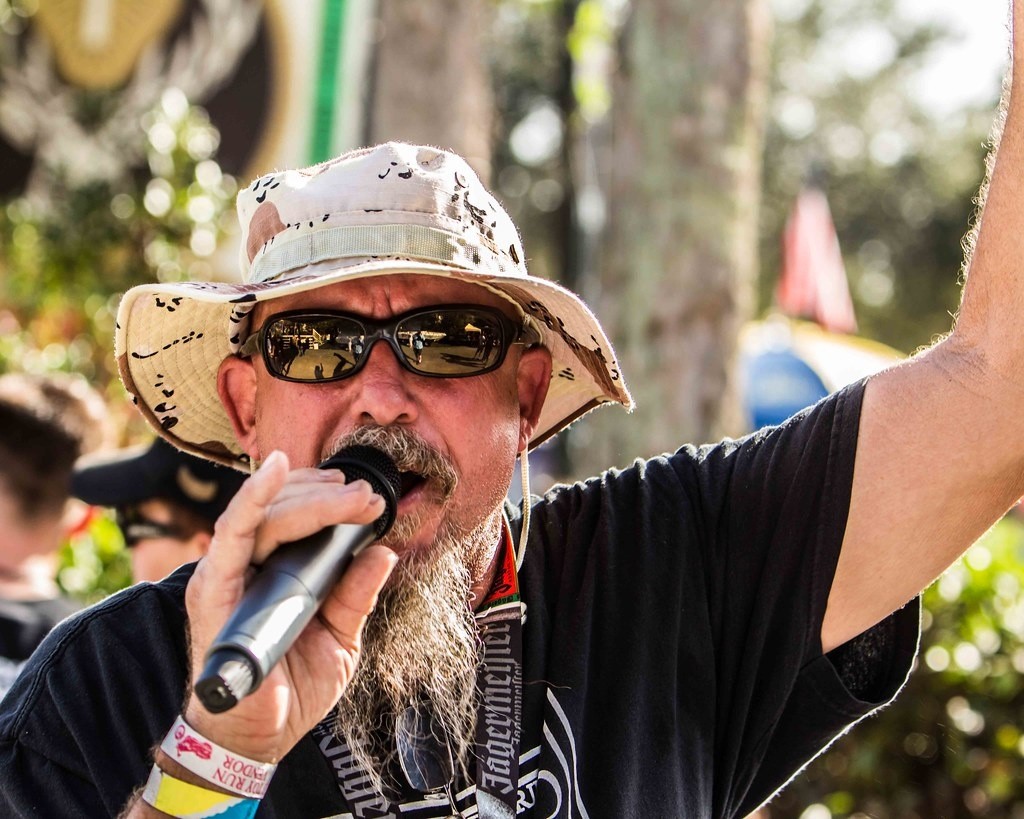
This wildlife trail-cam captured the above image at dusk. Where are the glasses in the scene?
[234,301,522,383]
[116,505,196,547]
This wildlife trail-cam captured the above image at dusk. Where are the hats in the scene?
[114,140,635,475]
[71,432,251,508]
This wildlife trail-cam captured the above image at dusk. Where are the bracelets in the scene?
[141,763,259,819]
[160,716,278,798]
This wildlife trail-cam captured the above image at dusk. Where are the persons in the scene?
[0,0,1024,819]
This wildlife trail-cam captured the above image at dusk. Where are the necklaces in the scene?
[313,510,524,819]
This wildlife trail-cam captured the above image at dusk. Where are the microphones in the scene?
[196,444,400,716]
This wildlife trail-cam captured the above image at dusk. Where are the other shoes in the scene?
[418,359,421,363]
